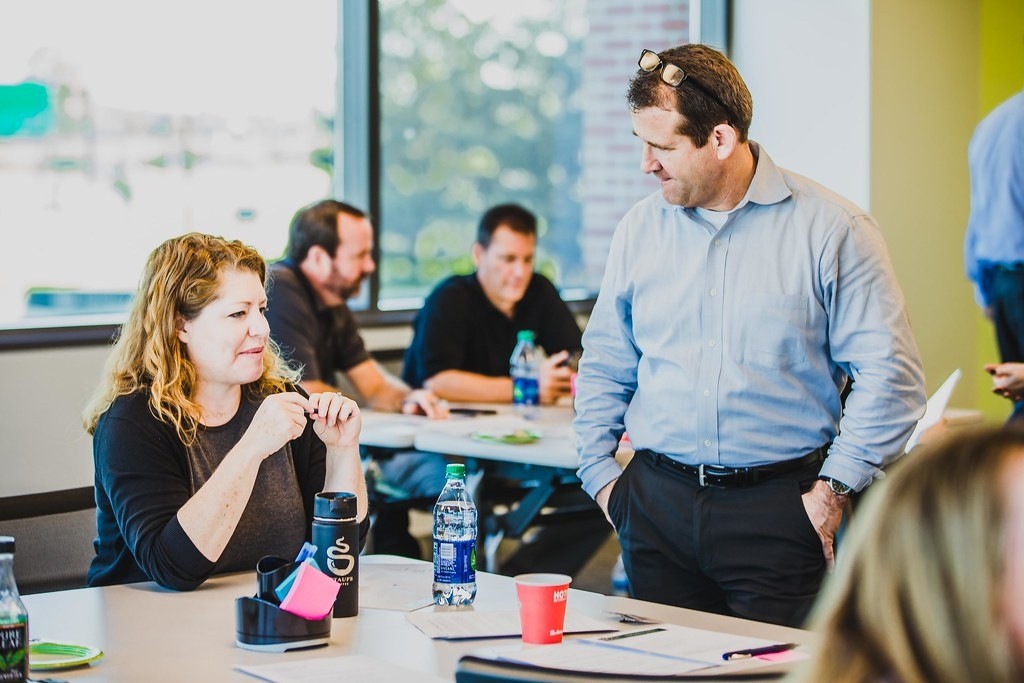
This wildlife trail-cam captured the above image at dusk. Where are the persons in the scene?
[571,45,930,628]
[967,90,1024,363]
[82,232,369,590]
[264,198,452,419]
[789,428,1024,682]
[379,203,609,580]
[984,360,1024,399]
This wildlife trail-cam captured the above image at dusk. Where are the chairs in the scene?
[1,484,97,598]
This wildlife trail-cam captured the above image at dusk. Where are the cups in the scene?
[513,573,572,644]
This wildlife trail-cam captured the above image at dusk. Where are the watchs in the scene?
[818,474,854,495]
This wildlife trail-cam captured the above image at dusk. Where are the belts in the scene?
[639,442,833,489]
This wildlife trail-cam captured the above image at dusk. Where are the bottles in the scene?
[0,536,29,683]
[433,464,477,606]
[312,492,359,617]
[509,330,538,419]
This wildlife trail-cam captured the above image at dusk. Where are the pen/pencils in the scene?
[304,408,353,416]
[722,642,800,661]
[448,407,497,415]
[295,542,318,561]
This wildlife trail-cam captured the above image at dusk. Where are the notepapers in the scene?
[277,557,342,621]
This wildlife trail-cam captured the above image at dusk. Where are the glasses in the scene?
[638,48,739,126]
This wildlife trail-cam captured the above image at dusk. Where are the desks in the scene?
[356,400,985,575]
[19,554,815,683]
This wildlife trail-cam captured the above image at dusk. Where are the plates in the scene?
[471,432,542,444]
[28,642,104,669]
